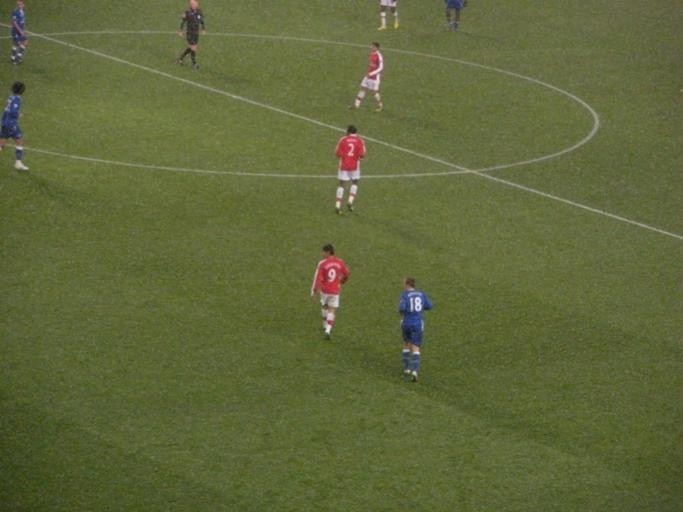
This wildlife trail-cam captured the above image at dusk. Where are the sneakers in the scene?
[447,20,459,28]
[11,54,23,64]
[347,102,383,112]
[404,368,418,381]
[336,202,354,215]
[14,159,29,170]
[177,58,199,68]
[324,330,330,339]
[377,22,399,32]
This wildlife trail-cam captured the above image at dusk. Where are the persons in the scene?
[346,42,385,114]
[398,276,434,383]
[308,242,350,340]
[0,80,30,171]
[333,124,366,214]
[174,1,206,72]
[9,1,30,66]
[440,0,468,32]
[376,0,401,32]
